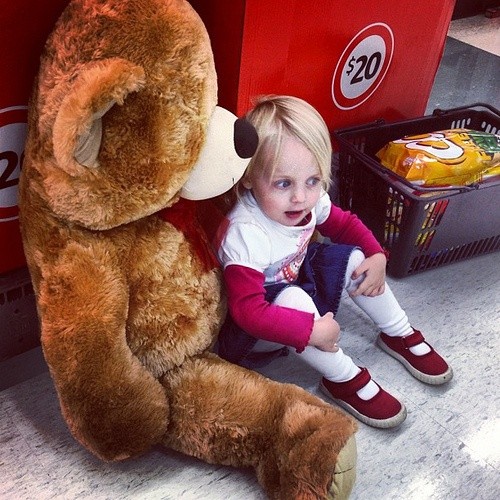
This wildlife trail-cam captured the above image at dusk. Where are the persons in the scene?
[211,94,453,429]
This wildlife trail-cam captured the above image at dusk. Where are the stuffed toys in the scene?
[20,0,359,500]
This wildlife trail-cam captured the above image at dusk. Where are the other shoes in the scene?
[319,366,407,430]
[376,331,454,385]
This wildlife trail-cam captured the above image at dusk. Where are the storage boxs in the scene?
[333,102,500,279]
